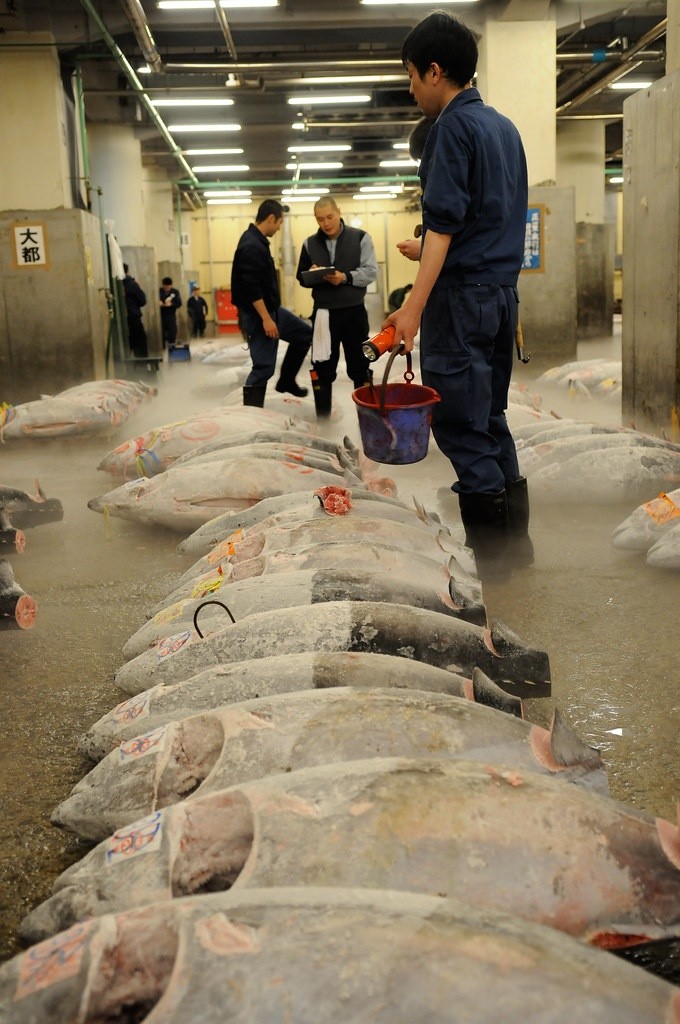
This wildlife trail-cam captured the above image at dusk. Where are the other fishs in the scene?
[436,357,680,525]
[0,379,159,445]
[0,405,680,1024]
[0,478,64,631]
[164,341,346,423]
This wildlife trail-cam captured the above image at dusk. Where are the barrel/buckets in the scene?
[351,343,441,465]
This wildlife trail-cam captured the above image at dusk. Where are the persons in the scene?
[388,284,413,312]
[230,199,313,408]
[380,11,534,582]
[159,277,182,349]
[187,287,209,338]
[395,117,434,260]
[296,196,378,427]
[122,263,149,358]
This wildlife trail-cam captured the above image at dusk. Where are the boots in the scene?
[244,385,266,408]
[275,344,309,397]
[309,370,337,418]
[505,476,535,568]
[450,481,512,591]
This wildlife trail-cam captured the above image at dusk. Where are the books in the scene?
[301,267,336,288]
